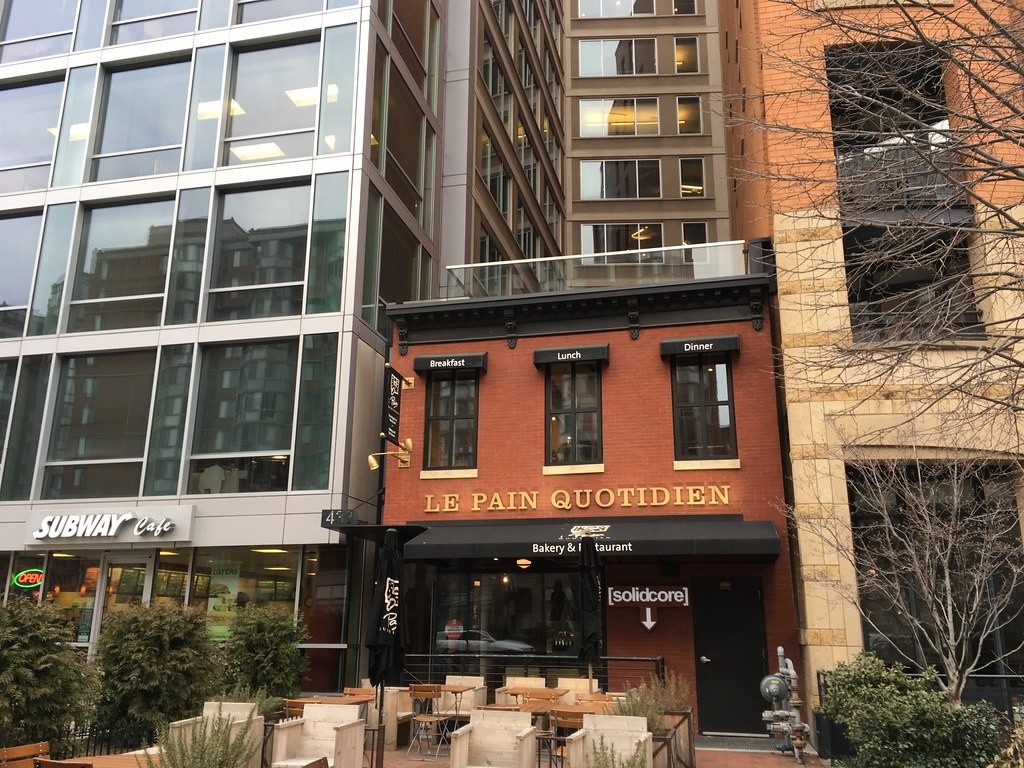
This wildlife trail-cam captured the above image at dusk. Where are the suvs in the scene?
[434,629,536,654]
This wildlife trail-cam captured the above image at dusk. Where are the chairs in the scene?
[1,668,656,768]
